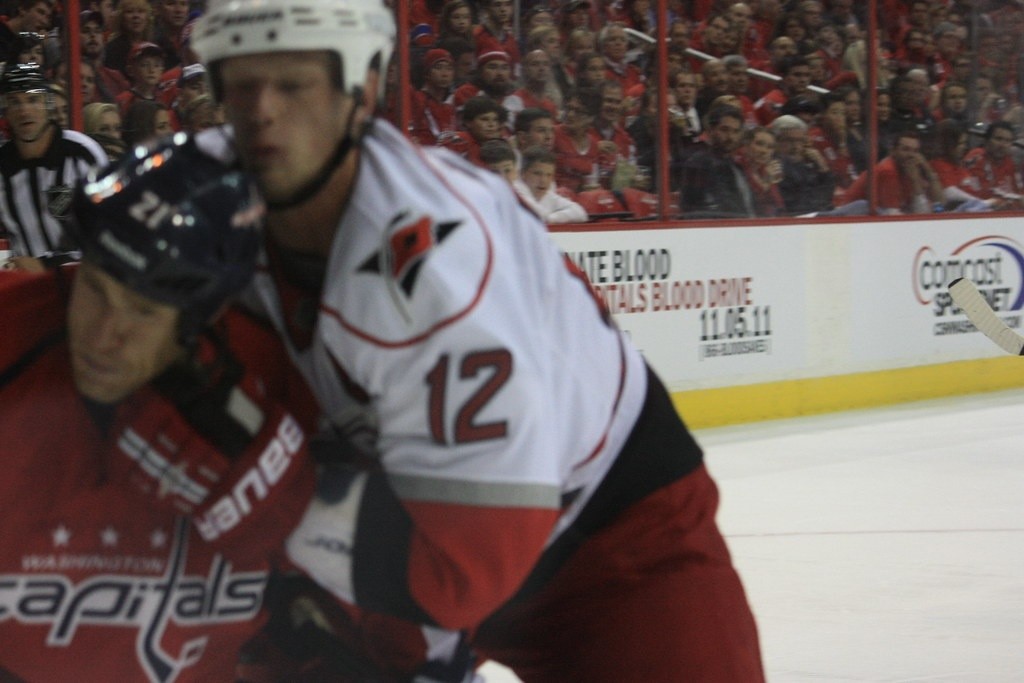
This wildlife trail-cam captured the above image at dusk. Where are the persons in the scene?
[0,0,1024,265]
[0,0,769,683]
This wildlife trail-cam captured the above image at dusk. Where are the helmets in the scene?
[0,62,48,96]
[191,0,397,98]
[66,143,261,312]
[18,31,46,42]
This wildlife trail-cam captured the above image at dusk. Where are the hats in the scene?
[562,0,591,12]
[128,43,160,64]
[79,10,103,29]
[475,29,519,63]
[181,64,206,79]
[422,49,454,71]
